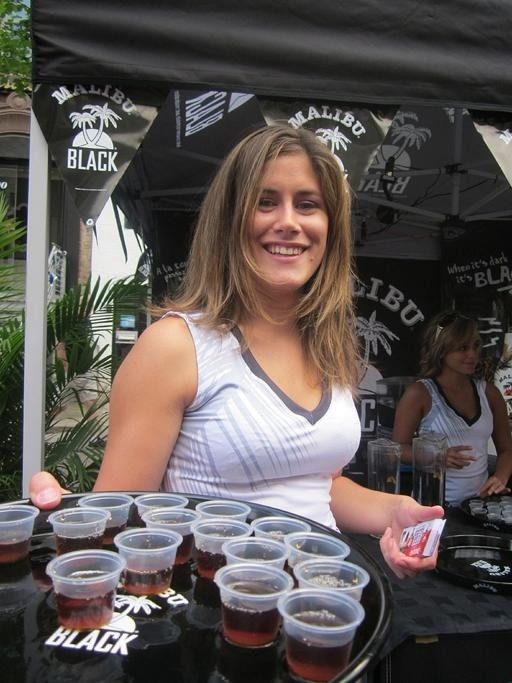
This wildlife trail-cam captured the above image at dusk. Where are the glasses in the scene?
[435,312,470,341]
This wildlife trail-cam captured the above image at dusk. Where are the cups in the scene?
[1,504,40,563]
[282,532,352,588]
[215,564,295,648]
[221,537,290,568]
[142,506,198,565]
[278,588,366,683]
[294,558,372,601]
[196,499,253,523]
[250,515,312,545]
[133,493,189,529]
[47,549,127,628]
[79,492,135,545]
[191,517,254,576]
[48,507,111,556]
[469,495,512,524]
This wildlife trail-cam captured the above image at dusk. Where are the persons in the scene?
[390,307,512,510]
[24,124,446,581]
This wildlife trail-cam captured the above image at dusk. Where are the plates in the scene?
[435,530,512,593]
[460,493,512,533]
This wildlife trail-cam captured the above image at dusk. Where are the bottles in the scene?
[409,431,447,508]
[367,438,400,538]
